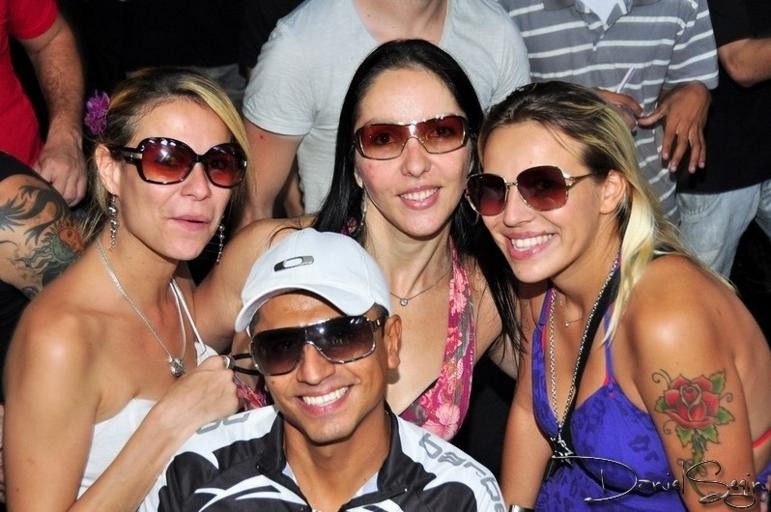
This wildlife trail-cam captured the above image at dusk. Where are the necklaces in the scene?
[355,189,452,307]
[547,252,620,433]
[93,227,188,379]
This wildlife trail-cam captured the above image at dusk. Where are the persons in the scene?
[132,226,506,512]
[231,0,532,227]
[2,61,253,512]
[1,151,84,375]
[496,0,720,226]
[0,0,90,209]
[673,0,771,281]
[471,78,771,512]
[192,38,511,439]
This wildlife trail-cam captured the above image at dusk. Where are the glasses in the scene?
[251,315,383,376]
[466,165,595,215]
[354,116,472,159]
[116,137,247,188]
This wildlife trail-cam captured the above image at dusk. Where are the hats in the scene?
[234,227,390,338]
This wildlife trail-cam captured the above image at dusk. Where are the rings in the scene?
[219,353,236,370]
